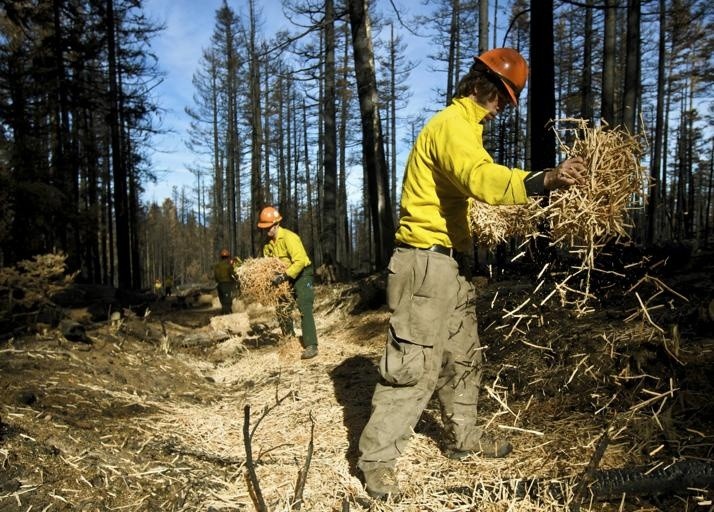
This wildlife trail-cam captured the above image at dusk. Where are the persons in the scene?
[356,46,591,500]
[154,279,164,296]
[230,255,243,269]
[258,207,318,359]
[214,251,237,315]
[166,276,173,296]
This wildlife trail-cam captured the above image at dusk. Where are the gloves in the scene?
[272,274,288,285]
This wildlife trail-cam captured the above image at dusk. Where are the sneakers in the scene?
[366,467,401,502]
[301,344,317,359]
[442,431,513,457]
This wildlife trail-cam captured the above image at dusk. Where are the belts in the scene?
[397,240,464,266]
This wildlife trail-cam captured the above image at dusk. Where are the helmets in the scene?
[221,250,229,257]
[474,47,528,107]
[258,207,282,228]
